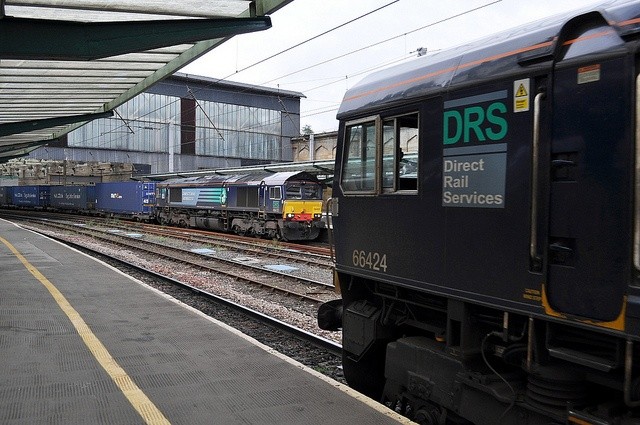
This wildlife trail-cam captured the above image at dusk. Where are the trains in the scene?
[318,0,640,425]
[0,170,323,242]
